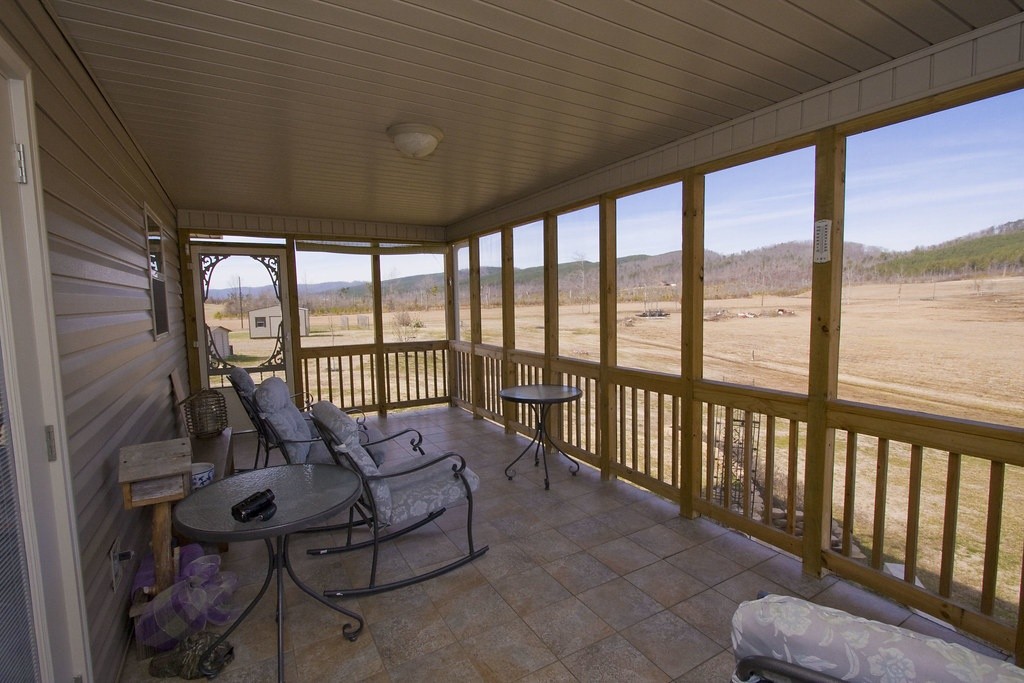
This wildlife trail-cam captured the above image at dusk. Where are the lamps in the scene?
[387,123,444,159]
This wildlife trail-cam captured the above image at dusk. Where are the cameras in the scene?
[231,489,277,523]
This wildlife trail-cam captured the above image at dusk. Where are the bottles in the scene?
[231,488,275,523]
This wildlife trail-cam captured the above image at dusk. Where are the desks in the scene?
[189,427,235,481]
[499,385,583,490]
[173,462,363,683]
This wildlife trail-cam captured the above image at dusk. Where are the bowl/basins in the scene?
[191,462,216,490]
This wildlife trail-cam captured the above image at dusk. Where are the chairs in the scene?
[226,365,489,597]
[731,591,1024,683]
